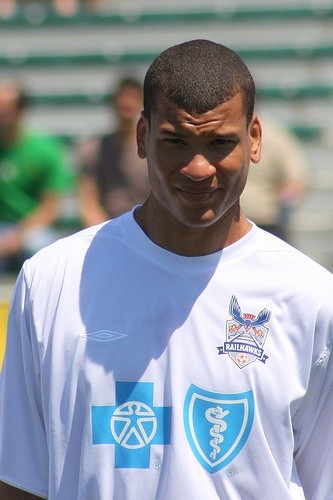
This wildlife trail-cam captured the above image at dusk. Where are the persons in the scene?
[0,38,333,500]
[0,77,309,274]
